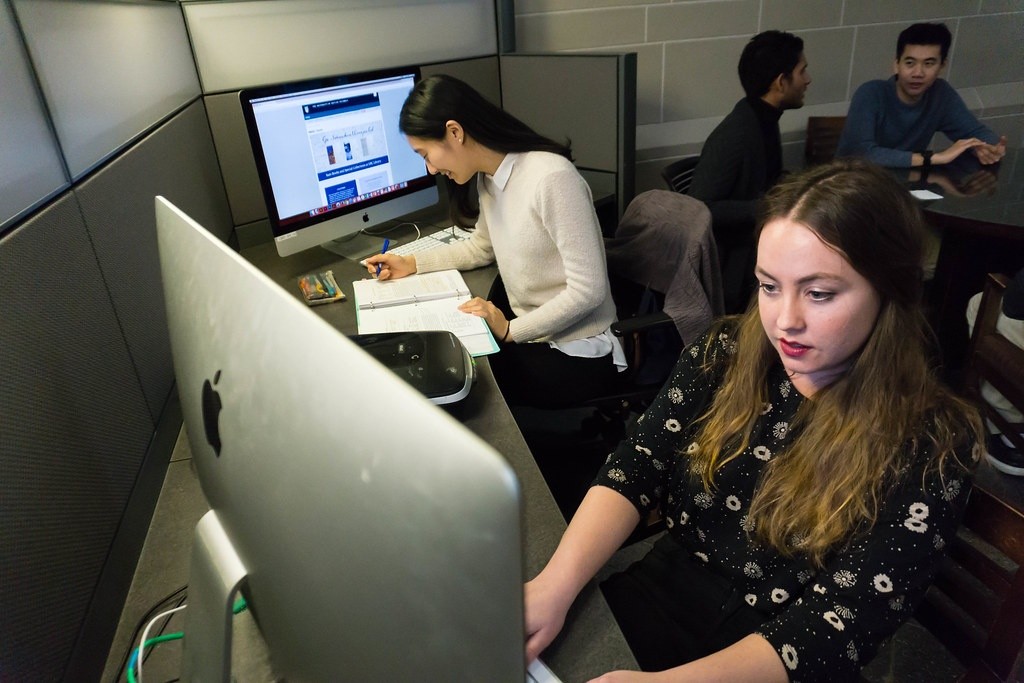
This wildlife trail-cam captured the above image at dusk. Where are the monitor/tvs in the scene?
[153,193,566,683]
[238,62,442,260]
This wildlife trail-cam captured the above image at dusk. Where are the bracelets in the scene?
[502,321,510,342]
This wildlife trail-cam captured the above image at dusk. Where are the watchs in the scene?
[919,170,930,185]
[921,150,934,167]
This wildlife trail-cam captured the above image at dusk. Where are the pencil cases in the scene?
[296,270,346,307]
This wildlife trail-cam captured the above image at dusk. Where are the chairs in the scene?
[850,275,1024,683]
[804,112,843,172]
[662,155,701,195]
[577,188,724,483]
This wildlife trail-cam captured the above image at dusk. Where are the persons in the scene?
[892,168,999,198]
[365,74,627,449]
[825,25,1007,167]
[686,32,812,224]
[965,267,1024,478]
[522,166,983,683]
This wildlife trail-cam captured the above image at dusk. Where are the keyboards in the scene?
[358,223,473,270]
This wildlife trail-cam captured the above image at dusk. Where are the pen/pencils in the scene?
[376,239,390,277]
[301,273,338,300]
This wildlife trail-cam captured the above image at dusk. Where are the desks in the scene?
[101,185,640,682]
[887,145,1024,242]
